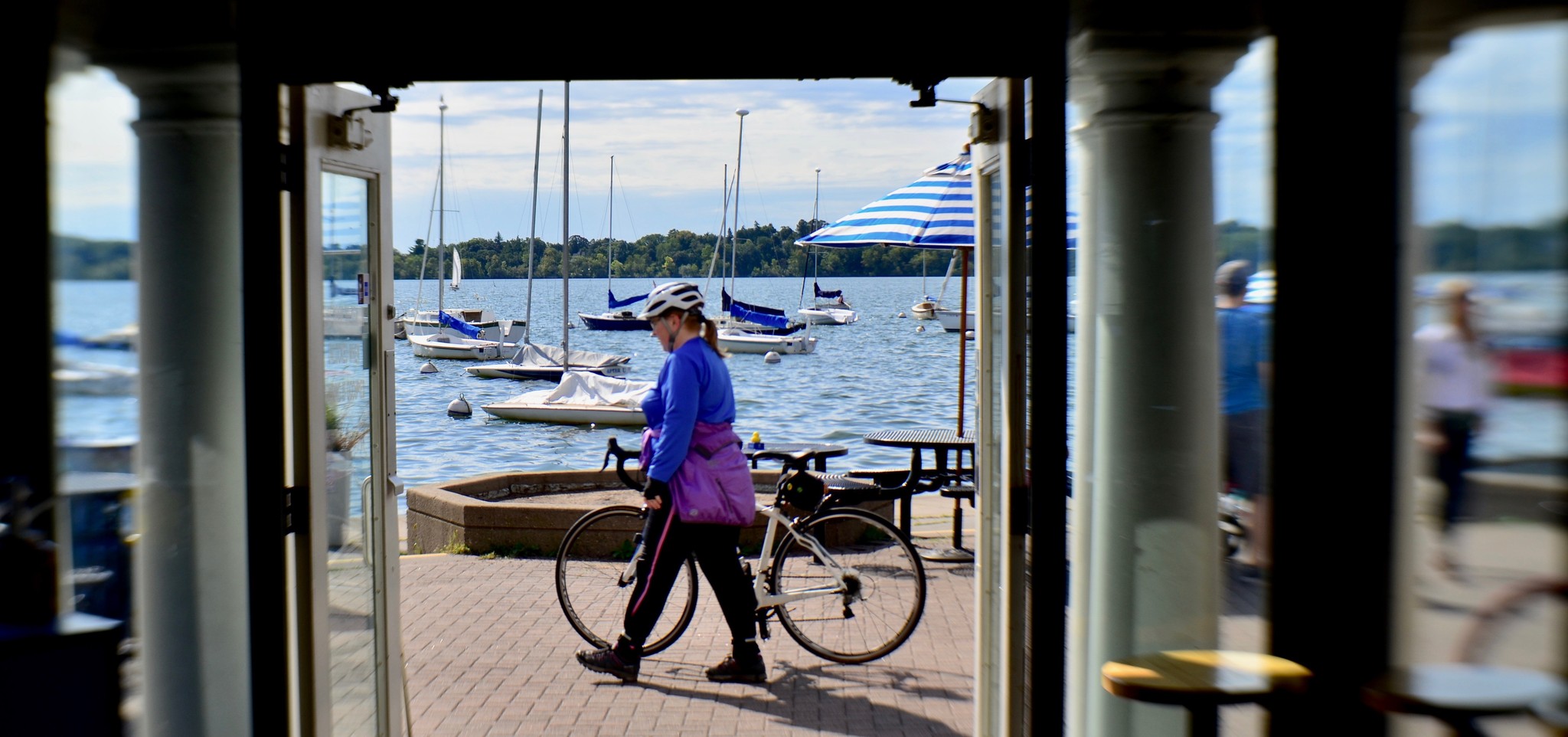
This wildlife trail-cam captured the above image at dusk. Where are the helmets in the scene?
[636,282,705,320]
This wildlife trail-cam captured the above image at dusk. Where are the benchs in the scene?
[814,466,977,566]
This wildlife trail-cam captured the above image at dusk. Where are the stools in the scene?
[1098,646,1309,737]
[1392,662,1568,737]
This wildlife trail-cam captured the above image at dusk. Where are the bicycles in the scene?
[553,434,927,665]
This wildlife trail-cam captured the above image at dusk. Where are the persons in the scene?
[575,280,769,685]
[1409,276,1493,532]
[1212,259,1272,572]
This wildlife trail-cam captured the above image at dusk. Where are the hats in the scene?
[1215,260,1252,284]
[1435,279,1476,305]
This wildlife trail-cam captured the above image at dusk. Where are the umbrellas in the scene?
[793,145,1078,511]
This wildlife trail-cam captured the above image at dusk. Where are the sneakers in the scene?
[575,644,640,681]
[705,654,767,684]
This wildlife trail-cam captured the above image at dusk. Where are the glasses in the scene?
[650,317,661,329]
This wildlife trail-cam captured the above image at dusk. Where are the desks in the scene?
[740,441,850,472]
[863,428,985,563]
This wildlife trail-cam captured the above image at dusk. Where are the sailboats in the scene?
[698,109,819,354]
[910,249,947,320]
[480,77,656,425]
[463,88,633,377]
[934,249,1078,337]
[577,155,654,332]
[392,94,525,361]
[795,169,857,326]
